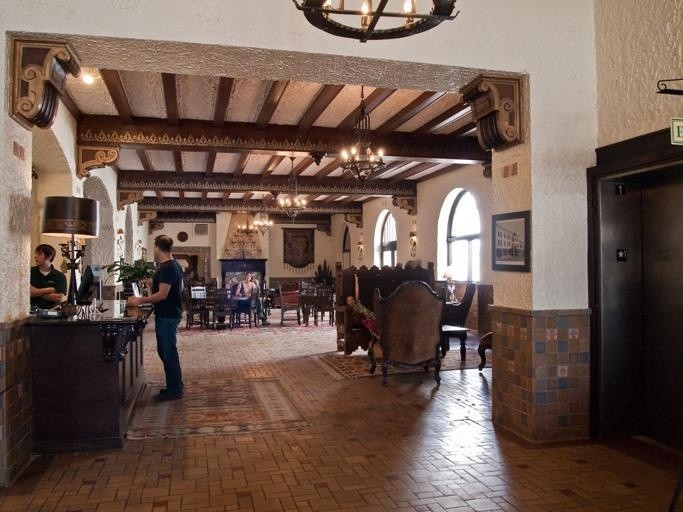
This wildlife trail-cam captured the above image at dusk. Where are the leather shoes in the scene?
[257,312,271,326]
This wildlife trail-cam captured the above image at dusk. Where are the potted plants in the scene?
[107,257,157,298]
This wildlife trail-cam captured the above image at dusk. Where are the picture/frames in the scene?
[492,210,530,273]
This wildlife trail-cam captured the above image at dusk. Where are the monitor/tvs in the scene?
[77,265,101,301]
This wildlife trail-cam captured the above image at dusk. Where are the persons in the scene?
[127,234,185,403]
[234,272,271,326]
[443,277,459,302]
[344,273,377,336]
[30,244,67,315]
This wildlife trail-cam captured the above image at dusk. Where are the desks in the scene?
[26,305,154,454]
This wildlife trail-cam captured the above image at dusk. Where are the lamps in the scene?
[278,156,307,224]
[40,195,100,305]
[339,86,385,191]
[237,212,258,238]
[254,194,273,237]
[293,0,460,43]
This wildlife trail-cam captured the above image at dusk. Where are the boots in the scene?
[151,376,185,401]
